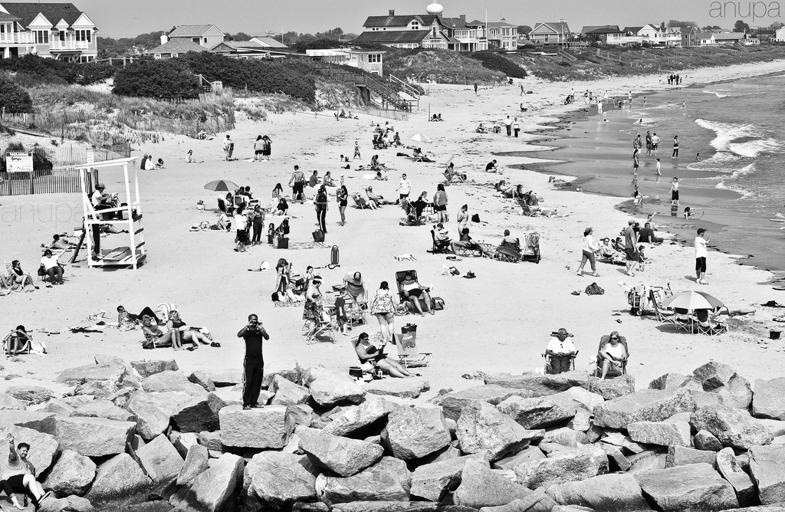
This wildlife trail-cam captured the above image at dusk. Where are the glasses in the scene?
[611,338,618,340]
[18,263,20,266]
[47,252,51,255]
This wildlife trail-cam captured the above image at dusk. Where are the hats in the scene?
[313,276,323,282]
[406,271,412,276]
[437,223,444,229]
[585,228,591,232]
[629,220,637,224]
[98,184,105,189]
[697,228,706,234]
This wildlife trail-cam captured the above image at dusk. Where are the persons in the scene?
[235,312,270,411]
[0,70,756,385]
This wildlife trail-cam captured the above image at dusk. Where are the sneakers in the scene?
[37,491,51,504]
